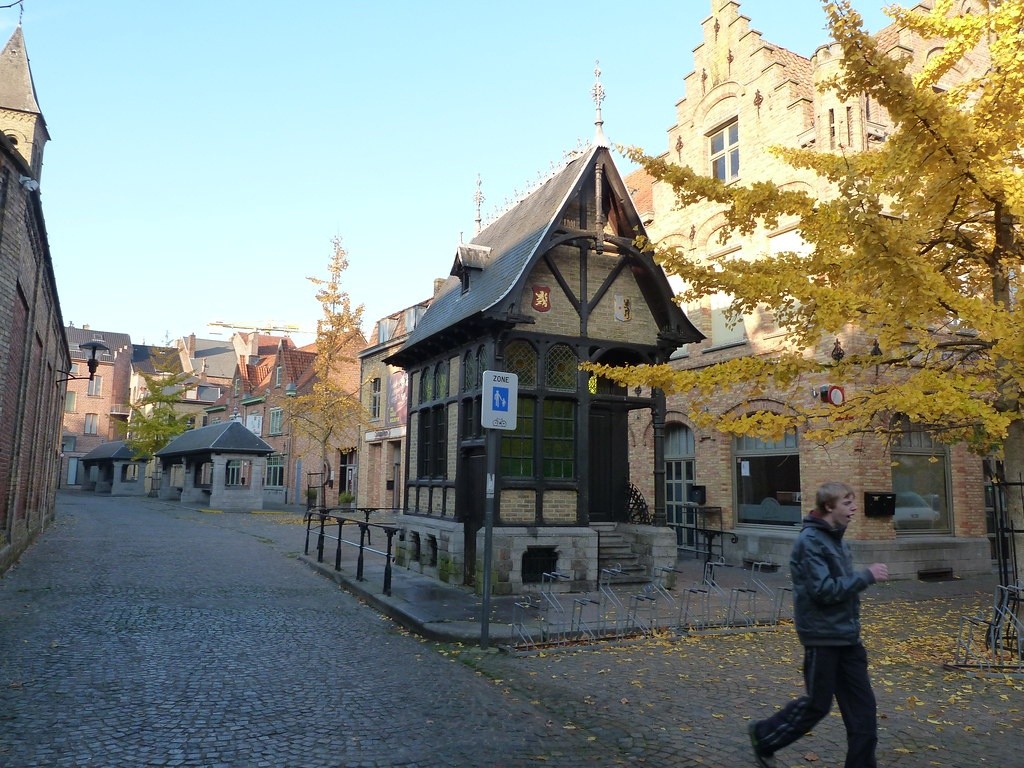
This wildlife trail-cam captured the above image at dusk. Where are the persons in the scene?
[750,481,888,768]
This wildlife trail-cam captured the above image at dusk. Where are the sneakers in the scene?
[750,717,779,768]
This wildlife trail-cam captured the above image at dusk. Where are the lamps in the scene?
[56,342,111,387]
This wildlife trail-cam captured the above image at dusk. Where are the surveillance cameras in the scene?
[20,174,39,191]
